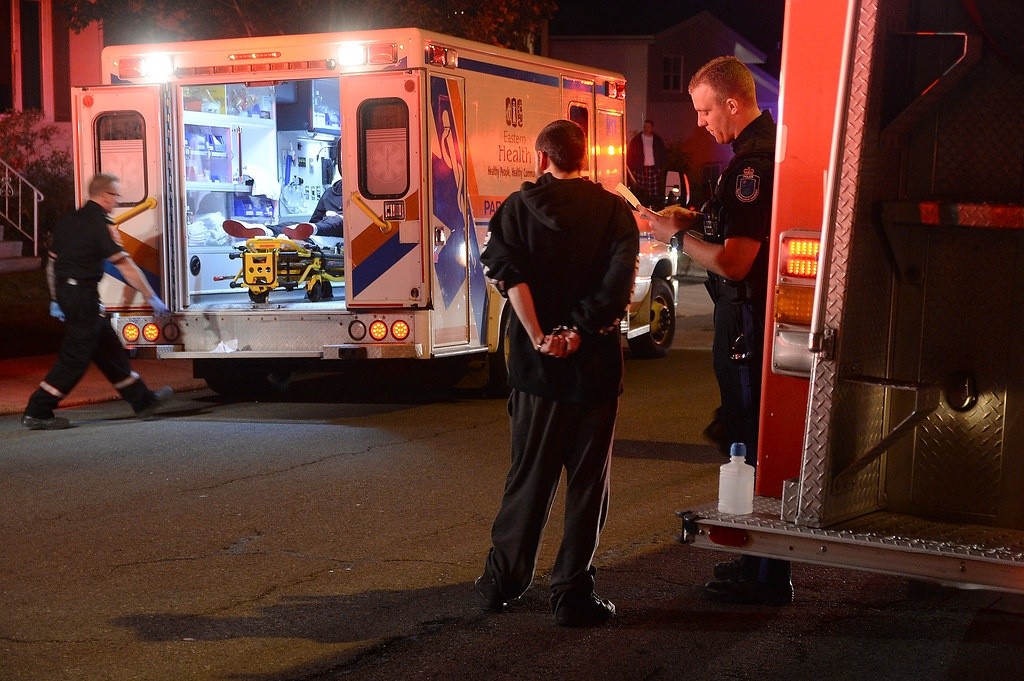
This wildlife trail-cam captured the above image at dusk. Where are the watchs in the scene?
[670,230,685,251]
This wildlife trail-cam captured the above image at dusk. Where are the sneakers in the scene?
[282,223,314,240]
[713,560,745,580]
[135,387,174,420]
[222,220,266,239]
[474,577,507,611]
[705,580,794,607]
[21,414,69,430]
[557,598,616,627]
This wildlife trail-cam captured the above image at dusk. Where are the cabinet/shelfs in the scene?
[183,77,343,296]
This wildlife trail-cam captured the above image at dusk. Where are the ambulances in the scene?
[672,0,1024,595]
[72,26,679,400]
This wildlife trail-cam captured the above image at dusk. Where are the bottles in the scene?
[315,91,323,112]
[718,443,755,515]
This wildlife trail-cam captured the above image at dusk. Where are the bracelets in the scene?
[537,341,545,352]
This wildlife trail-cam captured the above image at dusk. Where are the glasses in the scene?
[105,191,120,198]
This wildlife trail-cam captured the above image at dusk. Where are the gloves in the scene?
[148,297,170,316]
[49,301,66,321]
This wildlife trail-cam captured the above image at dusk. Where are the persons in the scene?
[668,185,679,205]
[223,179,343,238]
[629,120,668,214]
[473,120,640,626]
[635,56,793,606]
[22,174,172,430]
